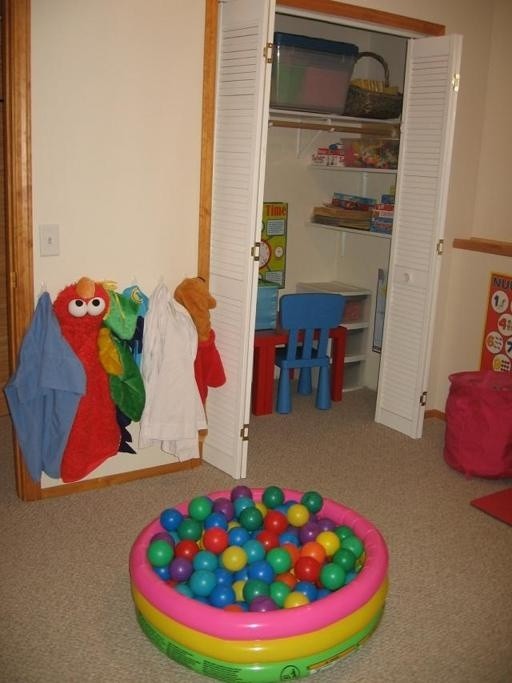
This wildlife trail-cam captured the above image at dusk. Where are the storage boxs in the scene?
[272,31,360,114]
[254,279,280,329]
[295,281,373,393]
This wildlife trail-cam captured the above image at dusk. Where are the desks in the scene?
[252,312,345,416]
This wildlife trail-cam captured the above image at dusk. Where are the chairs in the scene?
[274,293,350,416]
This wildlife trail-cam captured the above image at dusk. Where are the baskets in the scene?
[344,50,404,120]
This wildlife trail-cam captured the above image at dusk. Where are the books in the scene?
[312,182,396,234]
